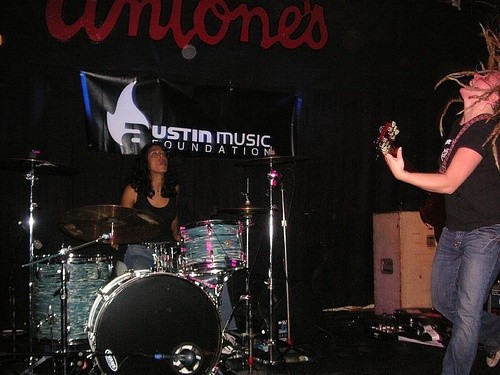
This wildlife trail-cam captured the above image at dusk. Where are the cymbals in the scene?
[219,204,269,211]
[9,157,57,165]
[57,204,172,245]
[242,155,305,161]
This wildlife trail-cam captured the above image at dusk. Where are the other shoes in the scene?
[486,349,500,368]
[221,333,249,355]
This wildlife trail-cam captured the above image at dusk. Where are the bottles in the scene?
[278,320,287,342]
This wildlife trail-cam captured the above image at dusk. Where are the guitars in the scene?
[373,120,447,243]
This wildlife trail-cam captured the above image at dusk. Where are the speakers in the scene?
[371,211,438,316]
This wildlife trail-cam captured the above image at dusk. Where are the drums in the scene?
[30,252,111,352]
[88,267,223,375]
[178,218,246,273]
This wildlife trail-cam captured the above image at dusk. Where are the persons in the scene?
[383,38,500,375]
[120,141,247,360]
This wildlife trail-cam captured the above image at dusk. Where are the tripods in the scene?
[222,220,270,375]
[18,237,100,375]
